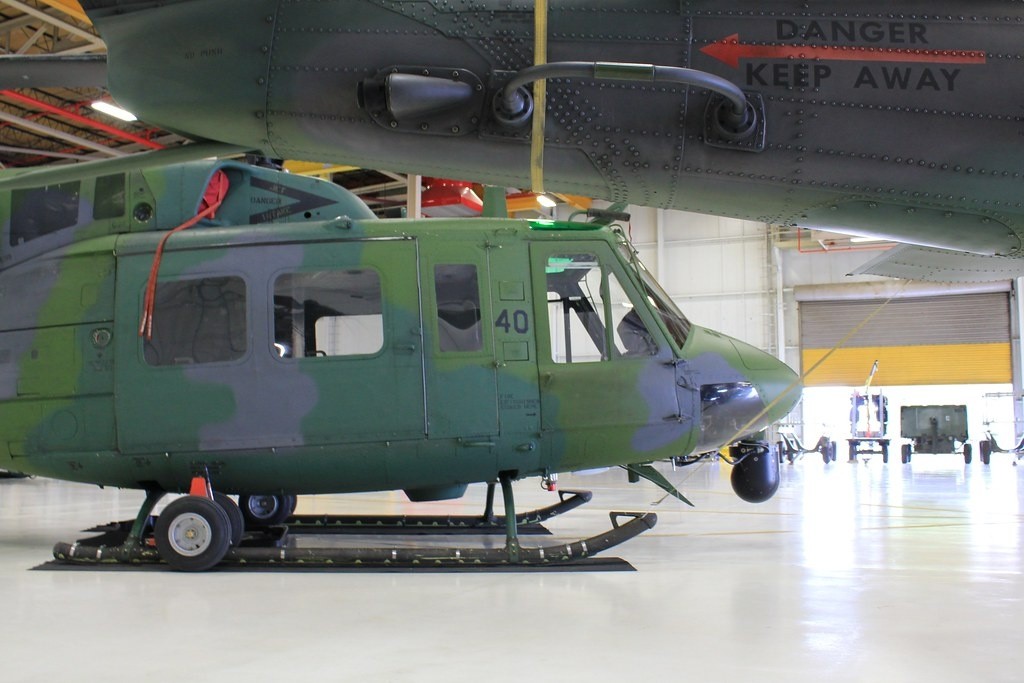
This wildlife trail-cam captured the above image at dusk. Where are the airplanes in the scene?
[3,0,1024,261]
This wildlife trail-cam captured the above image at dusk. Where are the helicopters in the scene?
[2,143,806,571]
